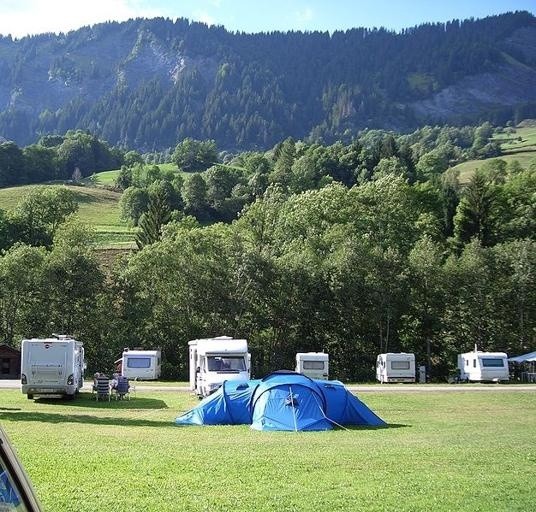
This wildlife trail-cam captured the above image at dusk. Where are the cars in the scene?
[0,412,45,511]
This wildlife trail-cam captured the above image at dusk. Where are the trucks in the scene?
[187,336,253,400]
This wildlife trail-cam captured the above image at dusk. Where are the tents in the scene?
[177,369,387,431]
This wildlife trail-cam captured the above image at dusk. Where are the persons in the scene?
[99,373,106,401]
[112,373,123,400]
[93,372,100,386]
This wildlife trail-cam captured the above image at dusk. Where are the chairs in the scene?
[92,376,139,402]
